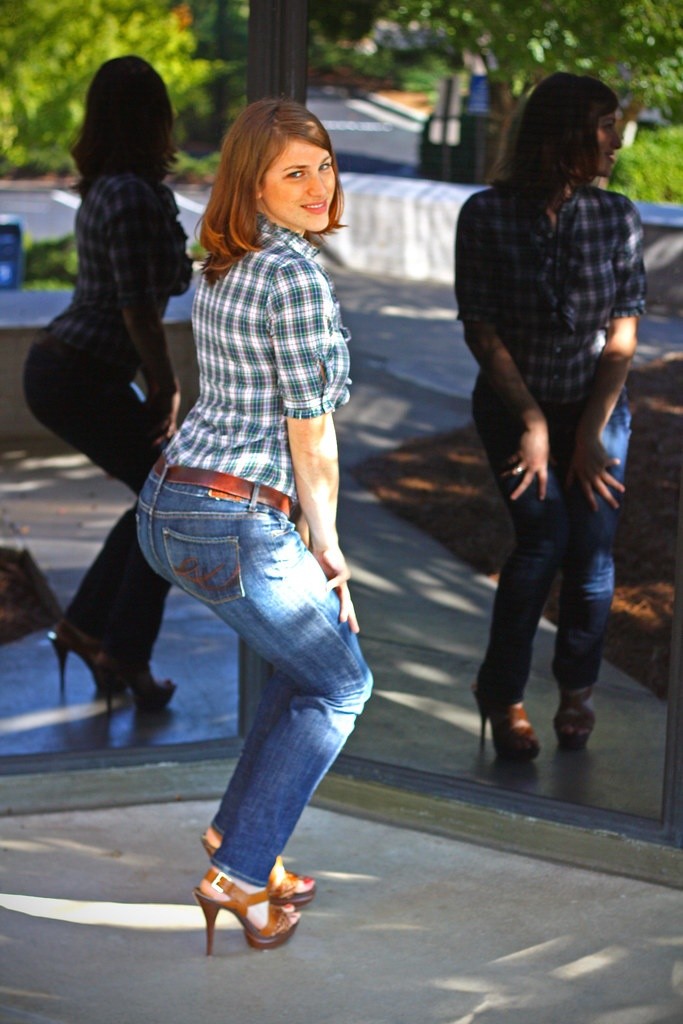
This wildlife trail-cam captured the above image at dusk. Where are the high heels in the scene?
[194,824,315,955]
[48,617,178,714]
[471,678,597,760]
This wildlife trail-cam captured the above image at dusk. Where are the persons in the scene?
[454,71,652,762]
[21,55,194,717]
[135,99,374,957]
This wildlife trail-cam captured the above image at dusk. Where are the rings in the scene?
[515,465,523,475]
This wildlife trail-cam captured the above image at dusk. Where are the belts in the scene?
[154,451,295,517]
[33,330,135,388]
